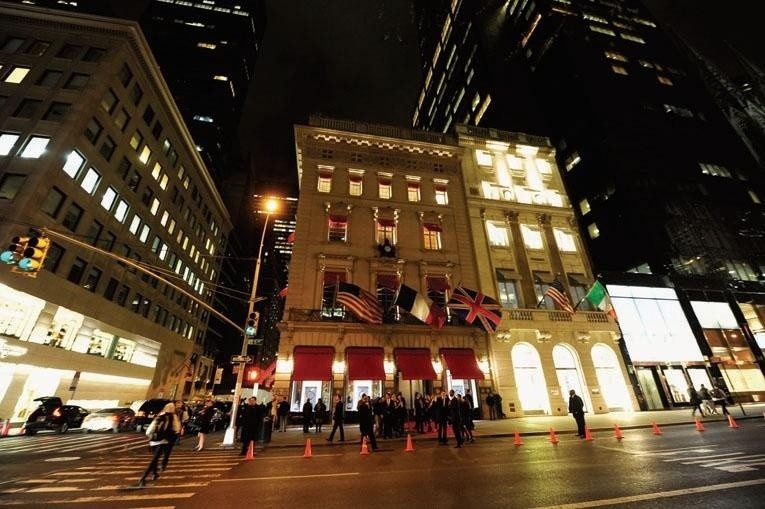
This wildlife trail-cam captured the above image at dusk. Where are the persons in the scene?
[314,399,326,432]
[414,391,439,434]
[436,389,475,448]
[688,383,707,418]
[711,383,730,416]
[137,402,182,487]
[486,390,502,420]
[357,392,407,452]
[195,400,215,451]
[236,396,261,456]
[303,398,313,433]
[569,389,586,439]
[272,395,290,432]
[699,383,720,416]
[326,394,346,442]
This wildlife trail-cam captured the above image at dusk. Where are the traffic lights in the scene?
[245,312,260,336]
[9,236,51,278]
[0,235,29,270]
[246,368,262,383]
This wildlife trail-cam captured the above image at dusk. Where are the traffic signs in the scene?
[246,339,264,345]
[231,355,255,364]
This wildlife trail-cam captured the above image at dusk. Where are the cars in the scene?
[192,398,232,433]
[80,406,136,433]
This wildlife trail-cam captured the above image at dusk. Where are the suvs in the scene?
[23,396,89,435]
[133,397,192,440]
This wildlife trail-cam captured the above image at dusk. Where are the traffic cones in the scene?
[613,423,625,439]
[244,439,256,460]
[694,417,706,431]
[0,418,12,437]
[403,433,417,452]
[652,421,666,435]
[727,414,741,429]
[547,427,560,444]
[512,430,524,446]
[16,424,26,435]
[301,437,314,458]
[359,435,369,456]
[583,424,595,441]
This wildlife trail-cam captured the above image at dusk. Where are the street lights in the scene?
[222,193,286,446]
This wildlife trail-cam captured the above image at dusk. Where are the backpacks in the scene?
[151,412,173,441]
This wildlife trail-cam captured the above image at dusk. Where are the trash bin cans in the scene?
[256,416,273,443]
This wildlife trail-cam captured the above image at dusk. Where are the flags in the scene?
[336,280,385,326]
[586,280,613,315]
[543,274,576,316]
[395,280,449,333]
[445,283,505,335]
